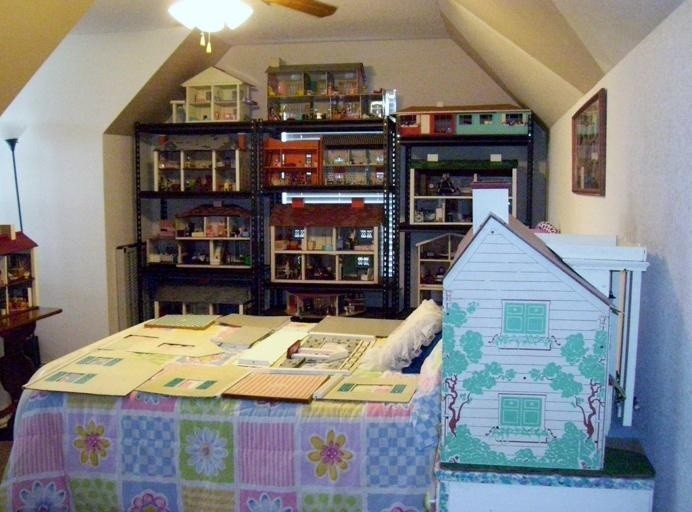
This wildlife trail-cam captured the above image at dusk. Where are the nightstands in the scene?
[426,438,657,511]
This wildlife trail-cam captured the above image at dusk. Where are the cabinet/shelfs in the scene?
[257,116,392,318]
[394,104,549,321]
[132,116,258,321]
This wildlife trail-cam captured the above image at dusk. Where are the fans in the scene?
[263,2,337,19]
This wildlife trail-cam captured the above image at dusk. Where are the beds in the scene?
[2,310,444,512]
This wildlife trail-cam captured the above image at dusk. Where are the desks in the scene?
[1,307,69,447]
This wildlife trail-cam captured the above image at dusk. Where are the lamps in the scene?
[166,2,255,55]
[0,119,26,234]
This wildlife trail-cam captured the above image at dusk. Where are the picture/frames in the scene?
[570,87,608,197]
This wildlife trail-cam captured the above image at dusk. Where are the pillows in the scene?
[371,294,446,375]
